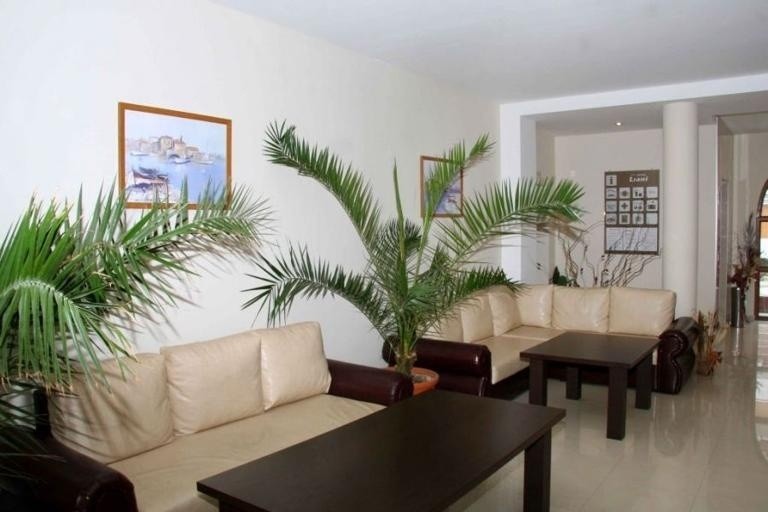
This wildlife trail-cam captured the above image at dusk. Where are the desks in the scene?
[196,388,568,511]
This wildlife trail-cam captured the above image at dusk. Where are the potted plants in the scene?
[241,115,598,374]
[727,210,768,323]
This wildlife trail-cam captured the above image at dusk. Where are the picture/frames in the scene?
[419,155,464,218]
[116,102,232,211]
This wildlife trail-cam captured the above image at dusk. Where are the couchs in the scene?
[2,323,413,508]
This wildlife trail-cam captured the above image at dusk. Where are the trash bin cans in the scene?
[730,286,744,328]
